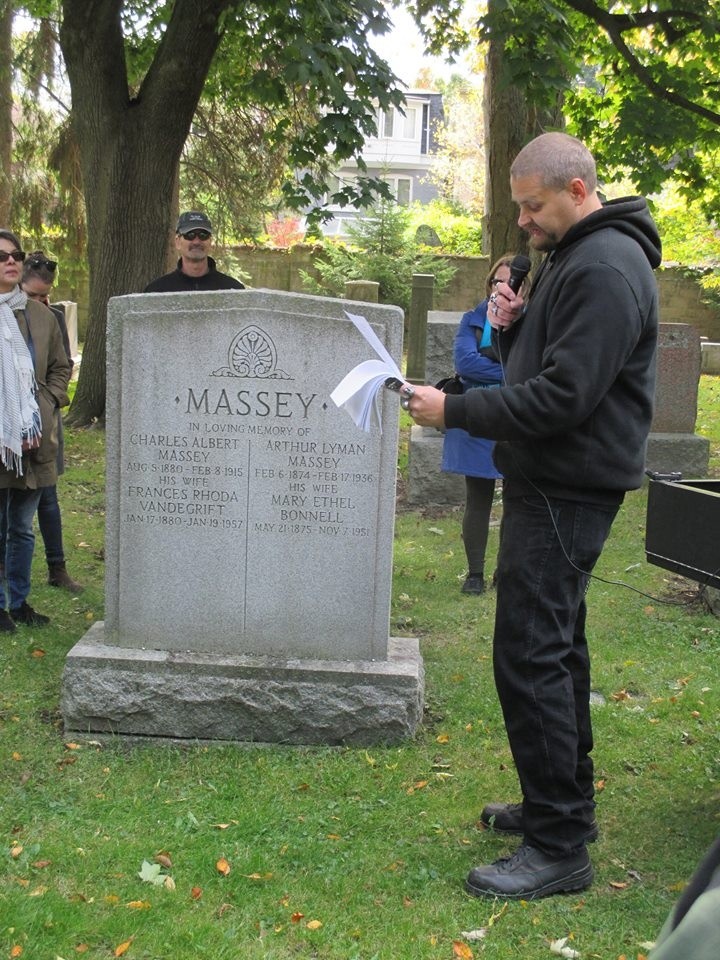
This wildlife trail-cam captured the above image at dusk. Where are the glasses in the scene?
[178,231,211,240]
[0,251,25,262]
[24,259,57,272]
[490,279,503,286]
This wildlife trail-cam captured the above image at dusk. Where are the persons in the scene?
[443,257,537,594]
[142,210,247,293]
[399,132,663,897]
[0,229,83,632]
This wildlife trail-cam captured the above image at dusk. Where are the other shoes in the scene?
[493,575,497,584]
[462,575,484,594]
[9,601,50,626]
[0,608,16,630]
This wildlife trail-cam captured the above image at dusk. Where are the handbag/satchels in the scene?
[436,378,464,395]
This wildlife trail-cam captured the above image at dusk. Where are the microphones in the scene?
[497,255,532,334]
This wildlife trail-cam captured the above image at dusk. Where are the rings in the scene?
[490,306,499,317]
[402,400,410,410]
[495,281,500,289]
[490,293,498,304]
[403,386,416,399]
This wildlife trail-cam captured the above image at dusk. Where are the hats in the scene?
[175,211,211,234]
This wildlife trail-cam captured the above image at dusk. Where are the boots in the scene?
[47,560,85,595]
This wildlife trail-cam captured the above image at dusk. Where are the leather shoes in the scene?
[465,843,593,900]
[480,802,598,844]
